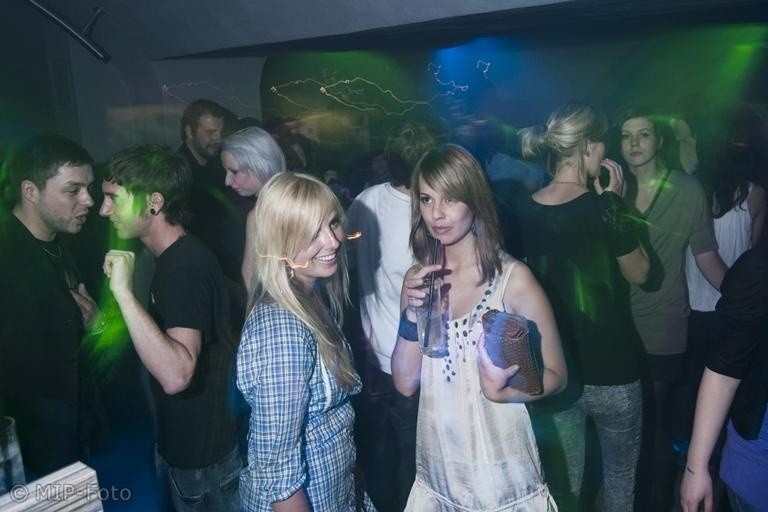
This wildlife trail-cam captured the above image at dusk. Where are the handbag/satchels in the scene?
[479,309,545,397]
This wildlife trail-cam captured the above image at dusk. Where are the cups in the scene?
[413,278,448,357]
[0,416,30,498]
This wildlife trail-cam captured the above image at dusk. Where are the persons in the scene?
[0,95,767,511]
